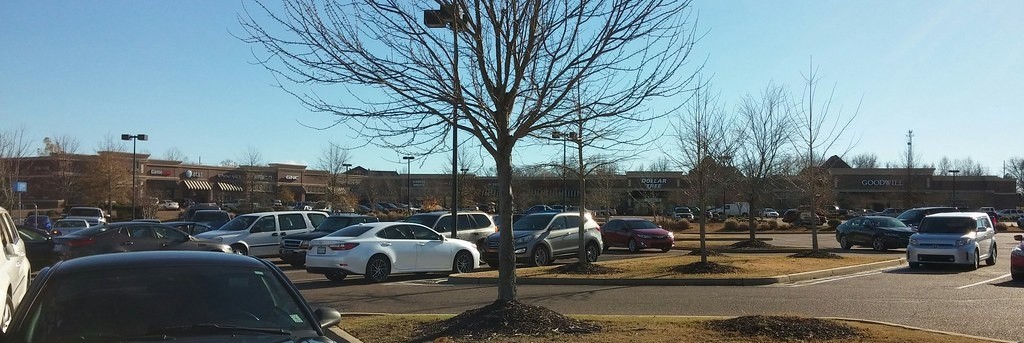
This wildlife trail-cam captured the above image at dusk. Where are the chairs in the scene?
[180,277,239,315]
[61,284,130,328]
[156,232,166,243]
[261,221,274,232]
[283,218,293,229]
[121,227,131,240]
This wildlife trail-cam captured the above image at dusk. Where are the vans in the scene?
[195,211,330,257]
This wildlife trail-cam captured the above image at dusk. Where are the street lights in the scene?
[403,156,415,211]
[342,163,352,187]
[121,134,148,220]
[551,132,578,212]
[948,170,960,206]
[422,4,470,239]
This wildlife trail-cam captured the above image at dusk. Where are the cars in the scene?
[673,201,1024,285]
[12,206,235,257]
[2,251,342,343]
[157,199,180,209]
[492,204,593,232]
[0,207,32,339]
[304,221,482,284]
[274,200,282,206]
[356,202,422,213]
[483,211,604,269]
[600,218,675,253]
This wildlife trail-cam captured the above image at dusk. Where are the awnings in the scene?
[327,187,346,195]
[183,180,211,191]
[486,189,500,197]
[217,181,244,191]
[532,189,581,198]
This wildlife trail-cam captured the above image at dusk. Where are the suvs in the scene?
[280,210,387,266]
[404,210,499,265]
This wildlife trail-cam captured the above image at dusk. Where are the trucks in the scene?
[711,202,750,219]
